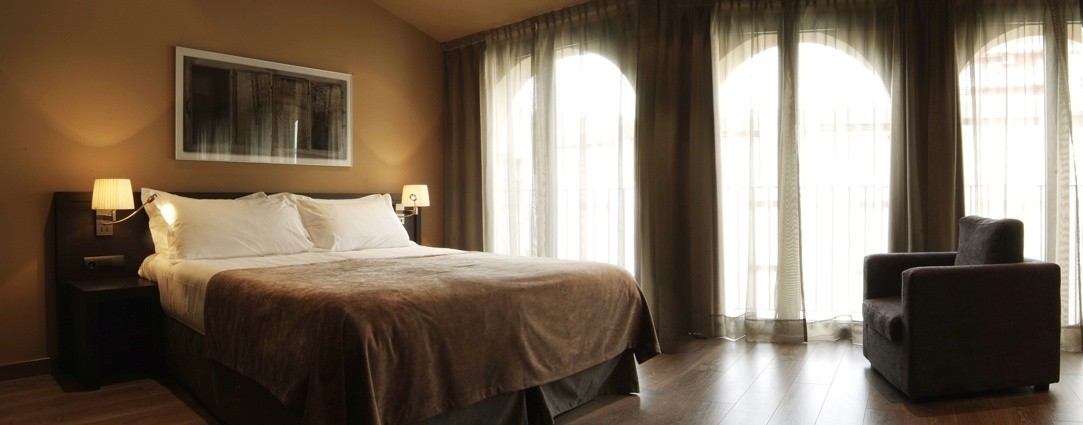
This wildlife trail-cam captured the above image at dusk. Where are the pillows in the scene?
[140,188,315,265]
[296,193,410,251]
[954,216,1024,266]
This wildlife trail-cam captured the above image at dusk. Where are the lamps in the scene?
[91,178,157,235]
[395,184,430,224]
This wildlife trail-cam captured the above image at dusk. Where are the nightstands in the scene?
[54,269,158,392]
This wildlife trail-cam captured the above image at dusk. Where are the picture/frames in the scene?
[176,46,352,168]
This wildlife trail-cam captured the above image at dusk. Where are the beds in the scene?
[54,192,661,425]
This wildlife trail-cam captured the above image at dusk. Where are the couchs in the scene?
[863,251,1061,402]
